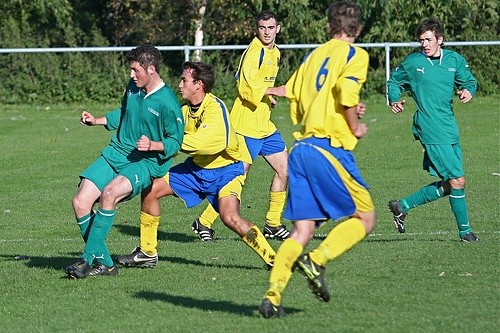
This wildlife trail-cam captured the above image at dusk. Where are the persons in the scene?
[388,19,481,244]
[65,46,185,279]
[259,0,375,320]
[110,62,295,279]
[191,11,291,243]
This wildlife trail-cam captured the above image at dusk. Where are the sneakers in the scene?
[263,261,274,271]
[263,224,291,241]
[114,246,158,270]
[88,265,118,276]
[191,218,215,242]
[388,200,406,234]
[290,252,330,303]
[258,298,285,321]
[65,258,90,280]
[461,232,480,243]
